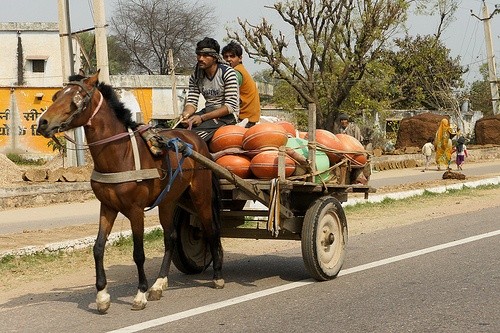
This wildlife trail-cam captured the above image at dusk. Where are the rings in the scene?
[195,121,198,124]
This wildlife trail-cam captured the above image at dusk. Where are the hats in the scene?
[339,114,348,122]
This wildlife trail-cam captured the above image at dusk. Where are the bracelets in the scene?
[199,115,204,122]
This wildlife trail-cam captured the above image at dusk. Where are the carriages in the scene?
[36,69,378,315]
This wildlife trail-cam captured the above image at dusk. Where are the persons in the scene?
[221,40,260,128]
[340,114,364,143]
[177,37,239,141]
[453,136,469,171]
[433,118,460,171]
[421,137,436,172]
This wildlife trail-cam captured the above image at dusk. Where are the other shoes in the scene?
[422,166,463,172]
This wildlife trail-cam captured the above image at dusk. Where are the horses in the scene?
[36,68,224,312]
